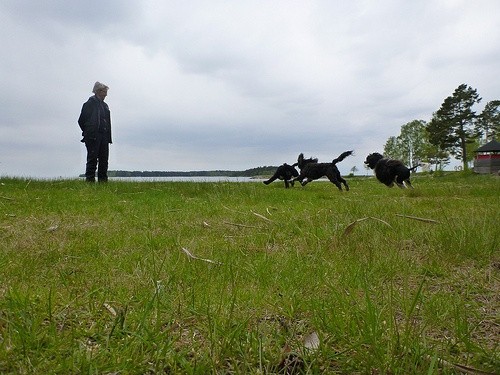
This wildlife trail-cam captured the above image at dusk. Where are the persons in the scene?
[78,80,113,182]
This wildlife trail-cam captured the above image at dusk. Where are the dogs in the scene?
[363,152,421,190]
[263,162,299,189]
[286,150,354,192]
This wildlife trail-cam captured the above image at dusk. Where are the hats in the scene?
[92,81,108,93]
